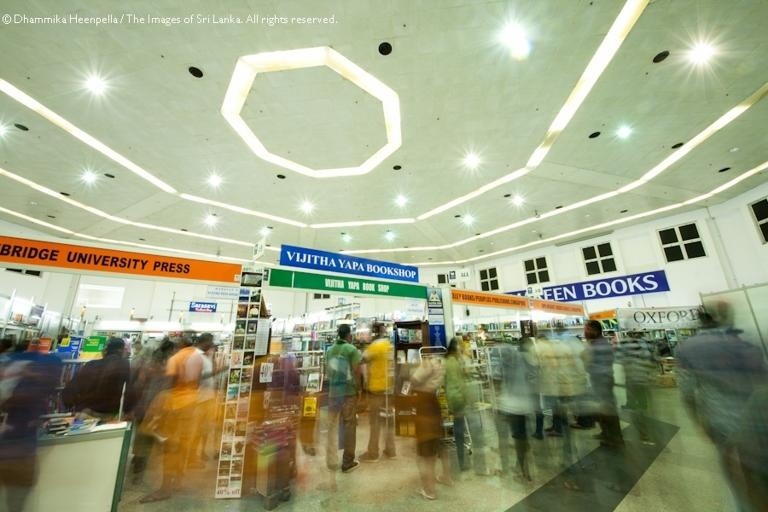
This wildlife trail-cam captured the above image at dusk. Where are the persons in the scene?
[326,322,361,471]
[677,300,768,512]
[411,336,493,500]
[0,331,229,511]
[357,321,397,462]
[497,320,655,494]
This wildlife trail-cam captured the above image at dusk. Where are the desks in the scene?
[0,414,130,512]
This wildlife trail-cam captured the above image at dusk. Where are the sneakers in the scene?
[342,460,360,473]
[358,450,398,462]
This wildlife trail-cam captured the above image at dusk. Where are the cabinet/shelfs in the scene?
[461,328,520,416]
[393,320,431,438]
[276,340,324,396]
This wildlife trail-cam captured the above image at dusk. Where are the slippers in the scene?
[416,487,437,500]
[436,473,455,485]
